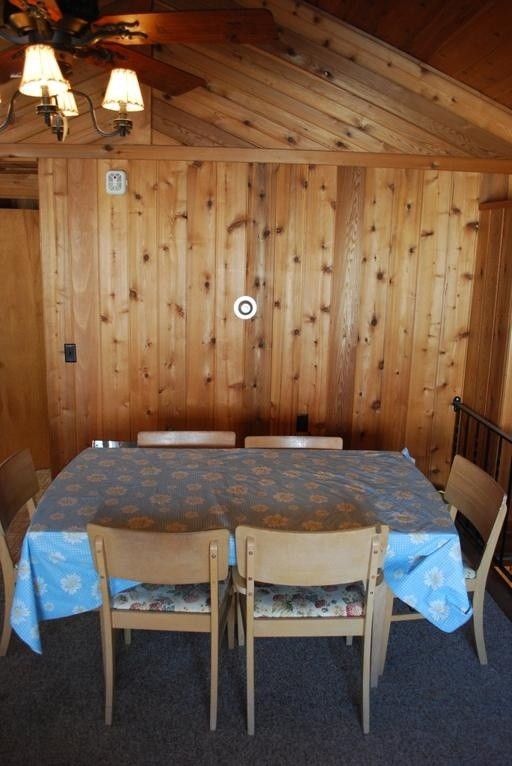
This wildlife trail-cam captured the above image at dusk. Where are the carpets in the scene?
[0,549,512,766]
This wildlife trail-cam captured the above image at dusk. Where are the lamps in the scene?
[1,0,154,145]
